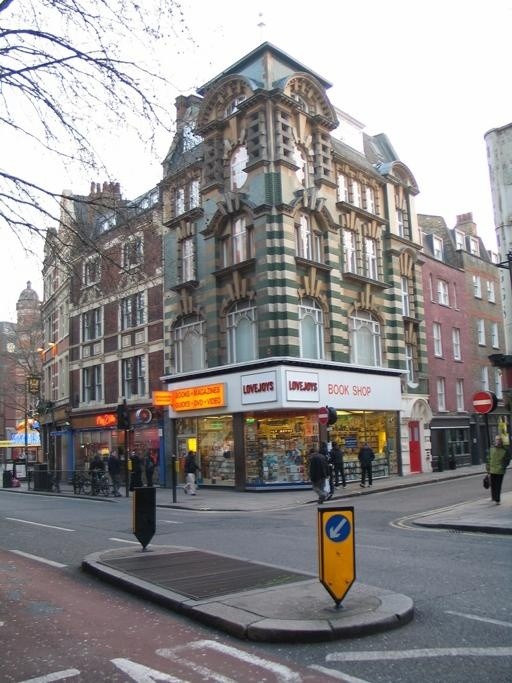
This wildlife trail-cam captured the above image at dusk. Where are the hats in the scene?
[494,439,502,447]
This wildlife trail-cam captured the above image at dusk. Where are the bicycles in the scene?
[72,463,110,497]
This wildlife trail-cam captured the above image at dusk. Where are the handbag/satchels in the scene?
[483,477,490,488]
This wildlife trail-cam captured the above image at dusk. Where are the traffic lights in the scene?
[117,405,132,429]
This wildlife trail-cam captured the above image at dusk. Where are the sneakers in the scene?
[335,486,340,489]
[368,485,372,488]
[360,484,366,488]
[318,492,332,504]
[343,486,347,488]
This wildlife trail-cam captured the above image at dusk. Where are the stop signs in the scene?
[472,392,493,414]
[318,406,331,426]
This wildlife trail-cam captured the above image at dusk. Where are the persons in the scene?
[129,450,140,488]
[358,443,375,487]
[309,448,333,504]
[183,448,204,494]
[485,433,512,504]
[88,455,105,493]
[107,449,124,497]
[317,449,336,494]
[145,450,156,487]
[328,440,349,489]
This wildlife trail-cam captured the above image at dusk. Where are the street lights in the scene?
[26,373,42,464]
